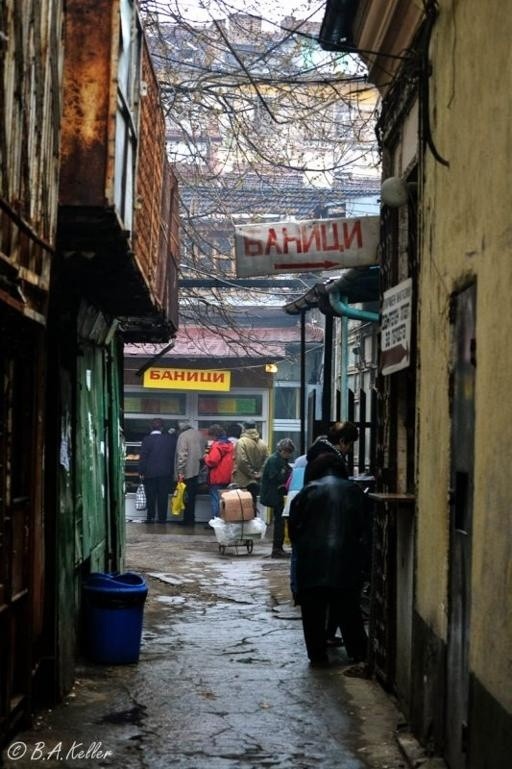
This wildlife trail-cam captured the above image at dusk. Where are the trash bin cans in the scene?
[81,572,147,665]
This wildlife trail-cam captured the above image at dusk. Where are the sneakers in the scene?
[178,520,196,527]
[144,518,167,525]
[271,548,291,558]
[342,662,371,679]
[309,635,344,669]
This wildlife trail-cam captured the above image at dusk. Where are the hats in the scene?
[243,418,256,428]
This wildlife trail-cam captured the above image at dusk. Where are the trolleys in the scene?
[209,481,265,555]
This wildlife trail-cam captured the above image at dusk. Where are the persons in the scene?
[286,451,372,669]
[224,423,241,445]
[137,417,177,525]
[302,418,361,648]
[231,418,270,519]
[259,436,298,560]
[174,418,209,527]
[285,453,309,548]
[203,424,236,530]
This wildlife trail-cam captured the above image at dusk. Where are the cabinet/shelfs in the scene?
[123,442,213,523]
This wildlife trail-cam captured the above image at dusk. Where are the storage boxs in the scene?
[217,488,254,522]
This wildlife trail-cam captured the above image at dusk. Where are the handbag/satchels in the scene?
[171,478,190,517]
[135,475,148,511]
